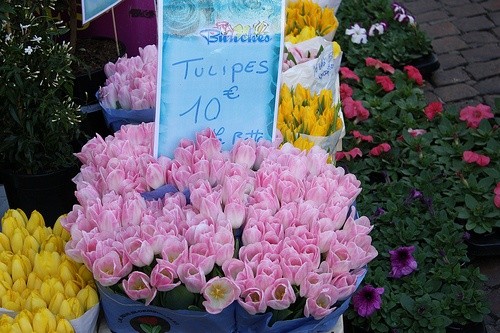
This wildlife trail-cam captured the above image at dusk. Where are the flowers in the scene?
[0,0,500,333]
